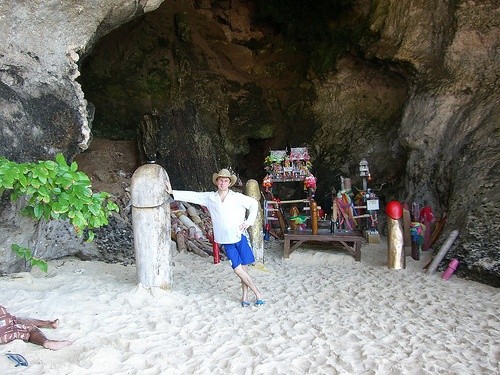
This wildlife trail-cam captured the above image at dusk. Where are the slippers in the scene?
[6,353,28,367]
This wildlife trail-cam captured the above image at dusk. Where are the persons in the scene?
[1,305,72,351]
[164,169,264,308]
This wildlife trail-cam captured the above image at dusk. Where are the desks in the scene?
[283,229,364,261]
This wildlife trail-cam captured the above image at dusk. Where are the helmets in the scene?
[385,201,402,219]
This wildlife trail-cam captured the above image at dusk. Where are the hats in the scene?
[213,169,237,187]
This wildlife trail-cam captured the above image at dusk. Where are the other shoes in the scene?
[242,301,250,307]
[255,300,263,307]
